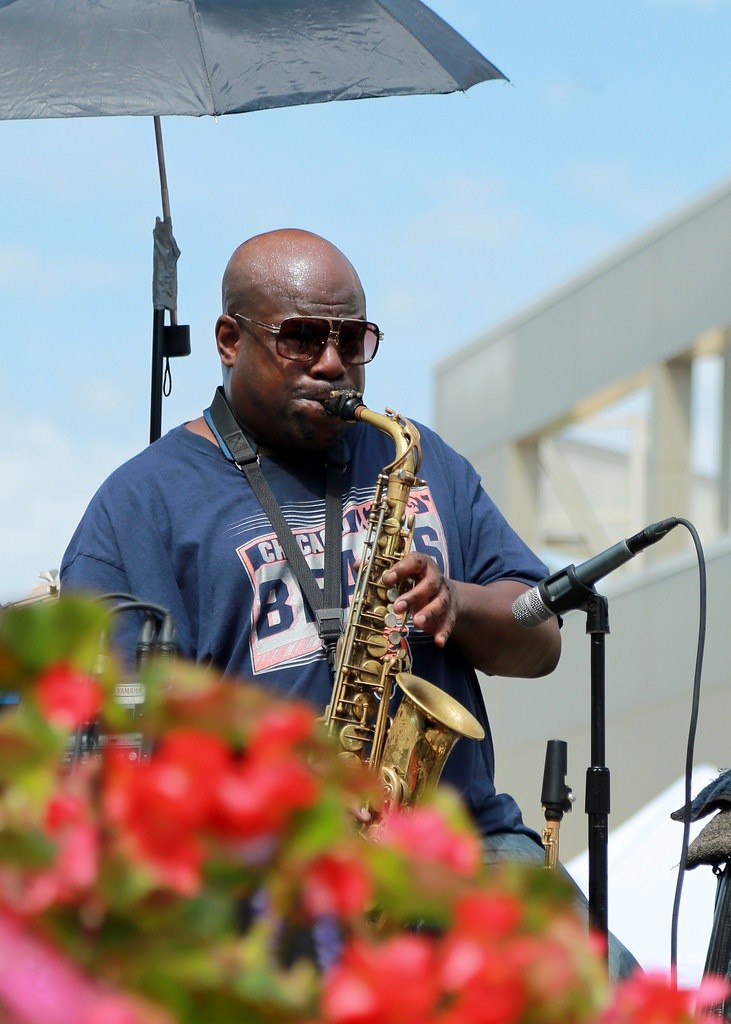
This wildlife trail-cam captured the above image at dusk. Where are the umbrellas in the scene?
[0,0,517,397]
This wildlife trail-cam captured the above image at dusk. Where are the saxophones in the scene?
[319,380,492,846]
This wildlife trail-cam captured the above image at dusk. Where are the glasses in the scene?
[231,313,384,365]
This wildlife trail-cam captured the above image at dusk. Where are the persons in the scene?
[60,228,650,992]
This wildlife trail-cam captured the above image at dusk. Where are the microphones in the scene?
[513,517,680,628]
